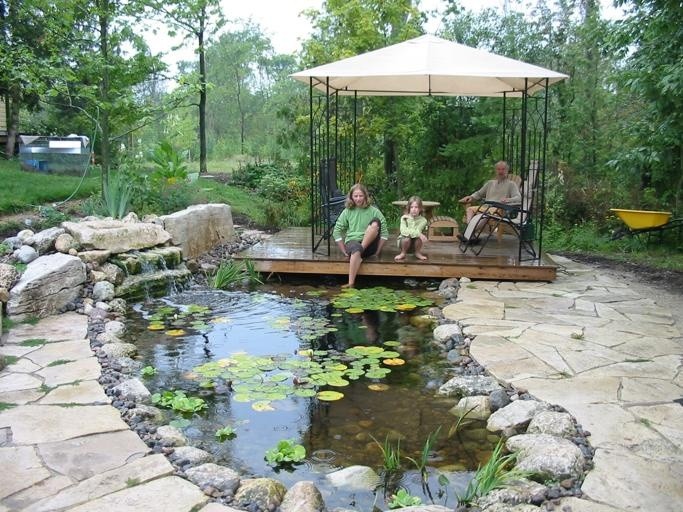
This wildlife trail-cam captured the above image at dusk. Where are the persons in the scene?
[390,195,429,261]
[454,160,523,247]
[331,183,389,288]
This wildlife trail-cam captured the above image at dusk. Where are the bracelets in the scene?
[343,251,347,255]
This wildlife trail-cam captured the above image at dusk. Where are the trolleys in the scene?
[609,208,683,251]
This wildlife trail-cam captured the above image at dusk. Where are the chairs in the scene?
[319,157,380,241]
[458,159,541,258]
[458,173,522,245]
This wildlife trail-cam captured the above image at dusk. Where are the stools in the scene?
[427,215,458,243]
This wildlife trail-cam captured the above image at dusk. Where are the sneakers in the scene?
[455,234,483,246]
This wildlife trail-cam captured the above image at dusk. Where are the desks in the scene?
[391,200,441,221]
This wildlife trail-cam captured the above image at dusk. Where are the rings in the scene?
[502,199,504,202]
